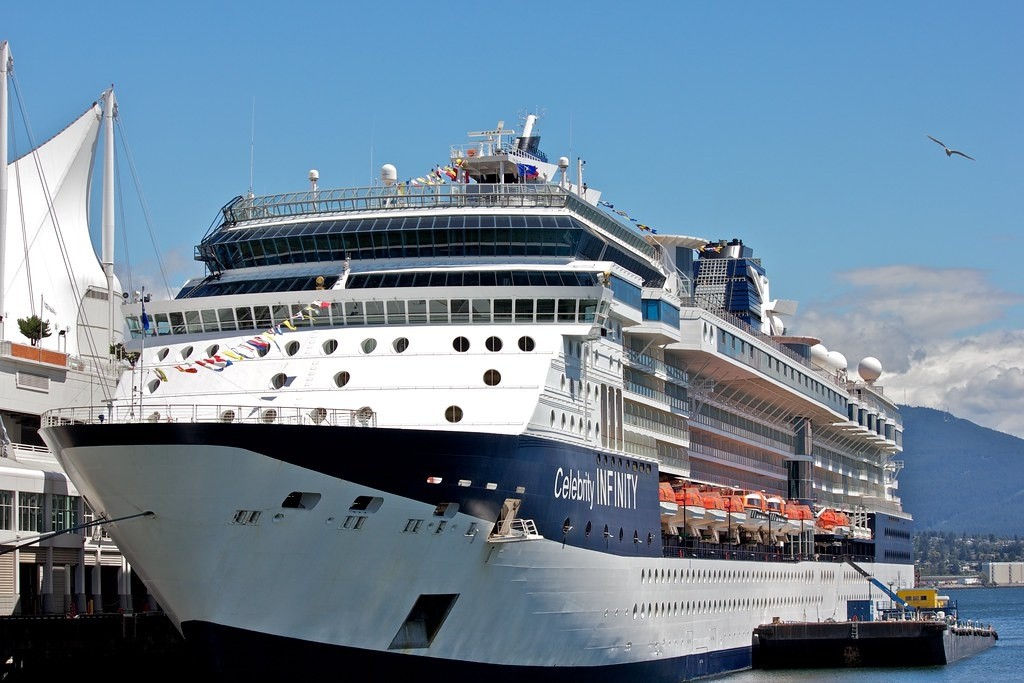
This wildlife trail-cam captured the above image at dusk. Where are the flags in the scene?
[695,245,722,254]
[396,164,457,196]
[141,303,150,331]
[516,162,539,179]
[600,200,658,234]
[152,300,331,382]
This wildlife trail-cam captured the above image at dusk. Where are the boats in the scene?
[658,481,679,524]
[720,490,747,531]
[815,510,839,536]
[794,504,814,533]
[698,485,727,524]
[836,512,852,536]
[787,500,803,535]
[764,493,790,533]
[671,481,706,526]
[736,489,769,530]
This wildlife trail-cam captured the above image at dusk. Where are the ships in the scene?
[37,110,919,683]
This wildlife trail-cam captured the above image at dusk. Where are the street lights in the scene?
[866,575,873,598]
[887,581,895,620]
